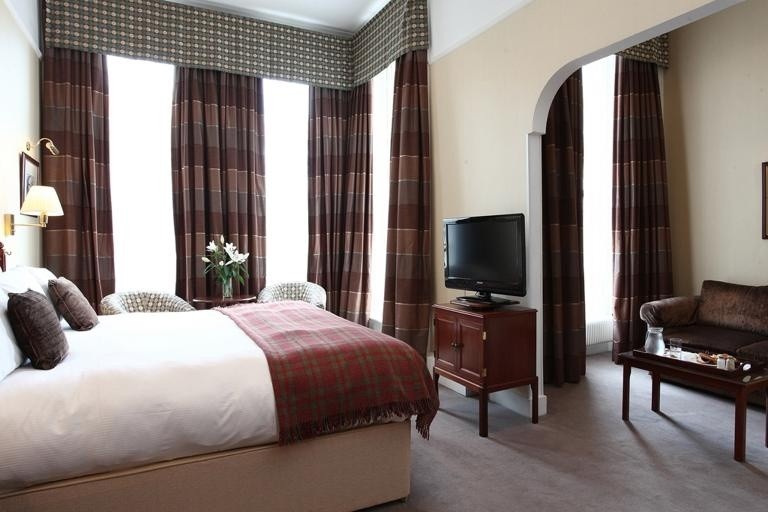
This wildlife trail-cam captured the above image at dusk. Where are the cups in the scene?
[669,351,681,360]
[669,337,682,352]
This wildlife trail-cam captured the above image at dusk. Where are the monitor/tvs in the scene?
[443,213,527,304]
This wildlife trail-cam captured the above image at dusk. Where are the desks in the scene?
[192,295,255,306]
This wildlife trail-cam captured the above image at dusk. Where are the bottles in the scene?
[725,355,735,371]
[717,355,725,370]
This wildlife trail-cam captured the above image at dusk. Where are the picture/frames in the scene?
[20,155,40,210]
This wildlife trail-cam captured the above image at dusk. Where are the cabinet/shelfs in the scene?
[431,300,538,440]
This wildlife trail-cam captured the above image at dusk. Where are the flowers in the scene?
[200,237,250,300]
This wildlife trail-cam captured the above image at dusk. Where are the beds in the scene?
[0,266,413,512]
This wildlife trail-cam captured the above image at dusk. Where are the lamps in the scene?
[26,137,60,157]
[4,185,63,245]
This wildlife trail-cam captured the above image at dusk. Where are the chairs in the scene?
[97,289,191,314]
[256,279,325,309]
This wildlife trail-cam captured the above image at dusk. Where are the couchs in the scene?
[639,280,763,382]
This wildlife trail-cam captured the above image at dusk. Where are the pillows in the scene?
[8,290,68,369]
[48,275,97,330]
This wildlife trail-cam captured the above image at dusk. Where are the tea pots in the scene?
[645,327,665,355]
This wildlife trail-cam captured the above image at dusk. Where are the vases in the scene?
[221,278,233,299]
